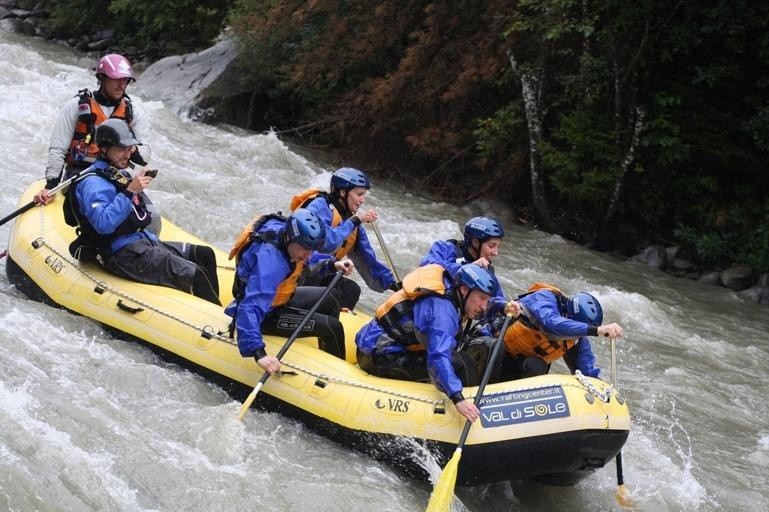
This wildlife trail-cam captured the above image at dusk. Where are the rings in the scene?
[469,413,475,417]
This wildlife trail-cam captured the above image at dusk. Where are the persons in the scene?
[288,166,402,311]
[66,118,222,308]
[476,283,625,379]
[34,54,161,237]
[418,217,507,301]
[355,263,521,423]
[229,208,347,376]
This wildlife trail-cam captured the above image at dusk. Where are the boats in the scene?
[4,179,631,487]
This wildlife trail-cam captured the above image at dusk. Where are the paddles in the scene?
[603,333,631,507]
[235,263,349,423]
[425,304,513,511]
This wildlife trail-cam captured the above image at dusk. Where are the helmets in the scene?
[330,167,370,197]
[452,264,498,297]
[567,291,602,326]
[463,216,505,245]
[95,118,143,147]
[95,54,136,83]
[285,208,326,250]
